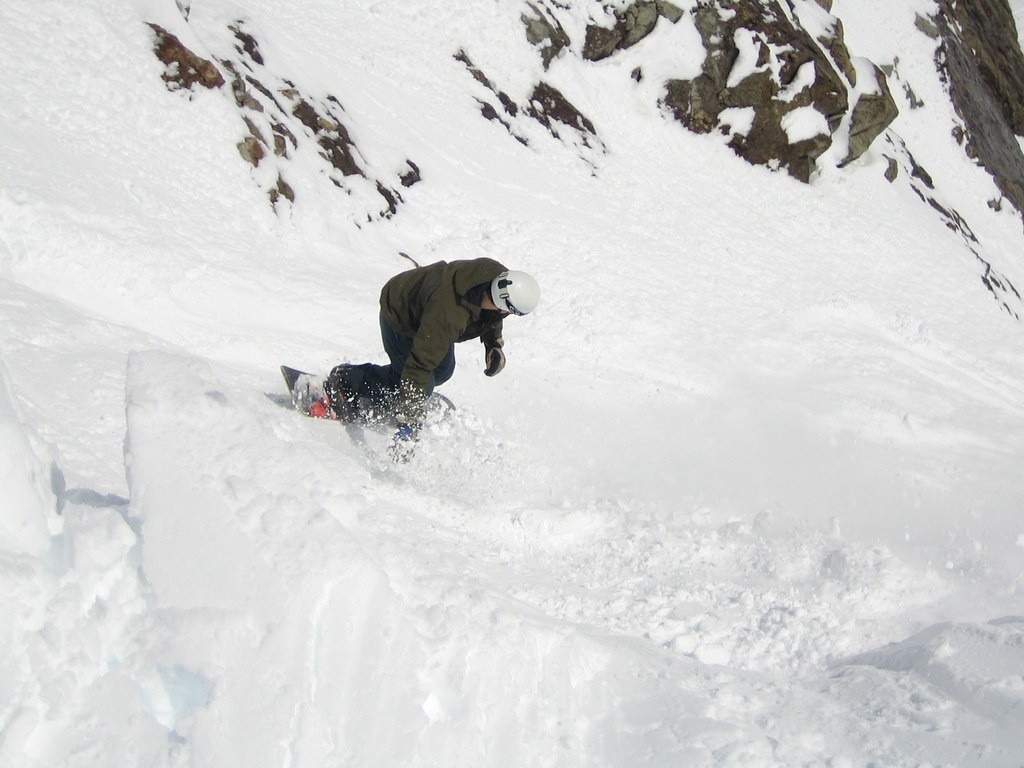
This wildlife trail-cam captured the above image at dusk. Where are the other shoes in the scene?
[323,363,360,424]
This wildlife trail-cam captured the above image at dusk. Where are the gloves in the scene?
[388,412,424,464]
[483,337,507,377]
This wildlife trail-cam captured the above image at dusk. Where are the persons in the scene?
[327,249,541,464]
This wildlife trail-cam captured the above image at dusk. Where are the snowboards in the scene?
[280,364,455,426]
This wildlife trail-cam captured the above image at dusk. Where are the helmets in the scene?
[490,269,540,316]
